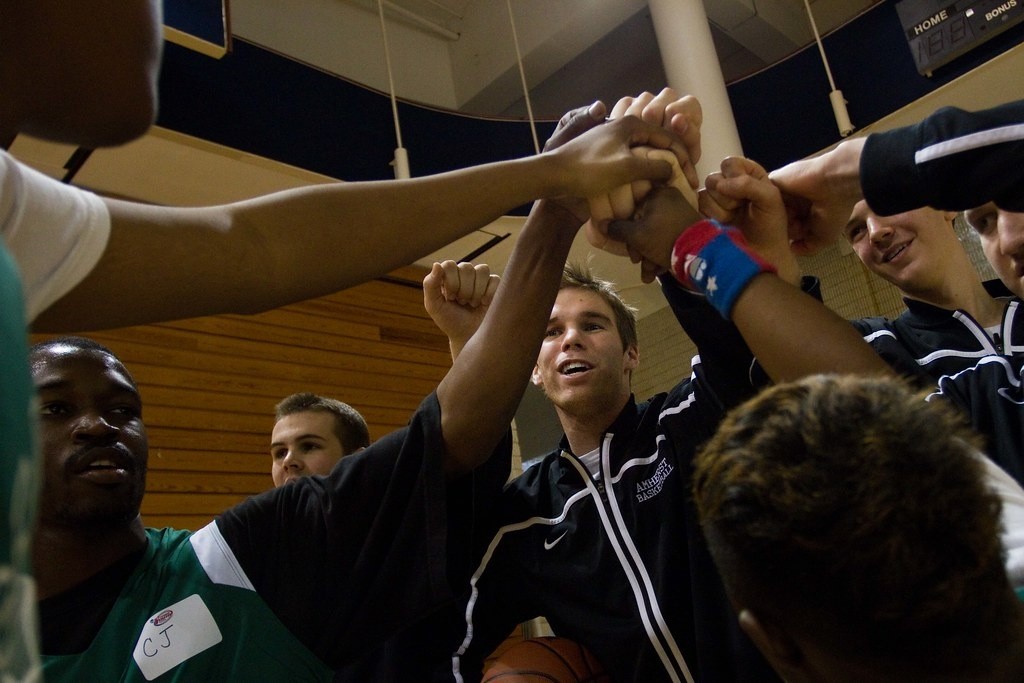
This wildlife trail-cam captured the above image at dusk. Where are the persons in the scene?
[0,0,1024,683]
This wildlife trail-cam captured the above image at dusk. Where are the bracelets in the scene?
[671,220,778,321]
